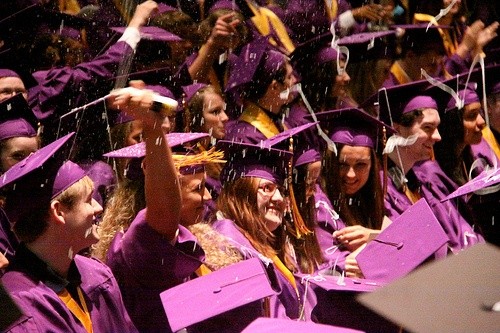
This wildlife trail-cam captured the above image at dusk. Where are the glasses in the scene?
[257,183,289,198]
[178,162,204,175]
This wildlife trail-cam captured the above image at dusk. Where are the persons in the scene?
[0,0,500,333]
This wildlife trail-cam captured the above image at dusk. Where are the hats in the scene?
[389,14,457,58]
[0,92,38,140]
[110,25,182,63]
[160,51,206,134]
[425,68,480,115]
[0,3,45,39]
[218,138,295,186]
[102,131,226,179]
[255,120,324,239]
[477,62,500,98]
[291,33,338,70]
[147,3,176,21]
[0,130,87,223]
[202,0,255,20]
[61,12,89,43]
[223,34,289,110]
[303,107,398,199]
[0,49,38,92]
[101,66,193,125]
[337,29,398,63]
[362,77,441,123]
